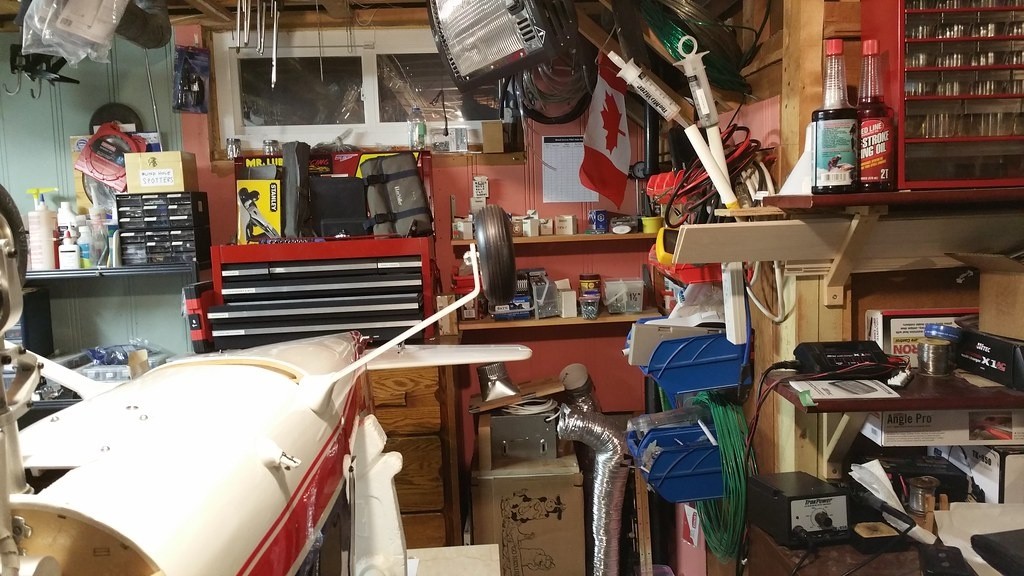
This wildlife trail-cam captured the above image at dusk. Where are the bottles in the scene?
[407,106,426,150]
[263,140,279,156]
[28,194,108,268]
[856,40,896,192]
[812,38,857,196]
[225,138,240,159]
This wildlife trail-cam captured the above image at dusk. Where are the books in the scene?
[237,179,281,245]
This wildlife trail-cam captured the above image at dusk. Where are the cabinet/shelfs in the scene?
[431,153,663,549]
[860,0,1024,190]
[760,182,1024,418]
[365,367,448,548]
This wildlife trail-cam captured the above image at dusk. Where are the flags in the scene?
[579,50,632,210]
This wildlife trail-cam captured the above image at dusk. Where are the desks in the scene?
[18,262,198,358]
[747,524,924,576]
[407,543,501,576]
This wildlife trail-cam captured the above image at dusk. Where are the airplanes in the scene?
[0,183,535,576]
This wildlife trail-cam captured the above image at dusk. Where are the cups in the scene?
[579,296,600,320]
[641,216,663,233]
[904,0,1024,137]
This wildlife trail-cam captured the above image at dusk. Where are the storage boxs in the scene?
[860,409,1024,447]
[944,252,1024,341]
[123,150,198,192]
[557,289,577,318]
[470,426,586,576]
[948,327,1024,393]
[554,214,577,235]
[864,307,979,368]
[481,121,504,154]
[523,210,539,237]
[540,218,553,236]
